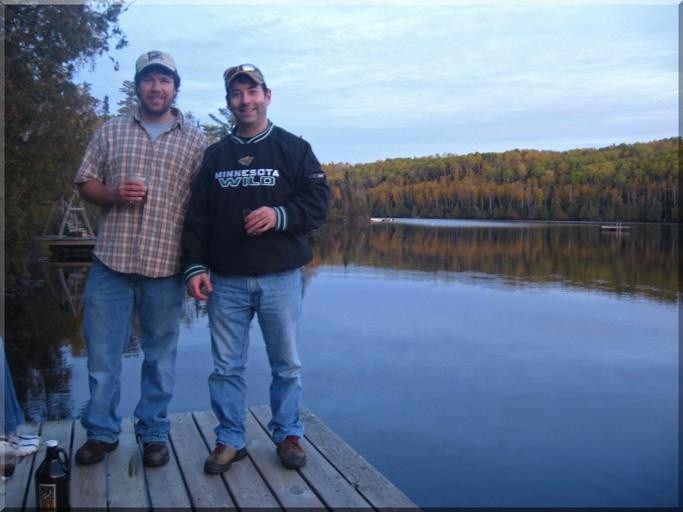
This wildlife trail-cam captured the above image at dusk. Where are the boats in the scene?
[601,226,632,230]
[369,218,385,223]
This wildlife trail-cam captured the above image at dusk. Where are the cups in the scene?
[130,176,149,204]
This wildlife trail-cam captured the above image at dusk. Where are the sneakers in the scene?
[143,441,169,466]
[277,435,305,468]
[0,434,40,456]
[76,439,118,464]
[205,443,247,475]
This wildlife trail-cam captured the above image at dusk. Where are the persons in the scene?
[74,52,211,468]
[180,64,330,474]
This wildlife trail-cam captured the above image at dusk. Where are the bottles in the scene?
[35,439,70,510]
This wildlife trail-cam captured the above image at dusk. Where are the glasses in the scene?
[224,64,256,81]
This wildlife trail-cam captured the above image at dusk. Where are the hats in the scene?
[135,51,176,73]
[225,64,264,89]
